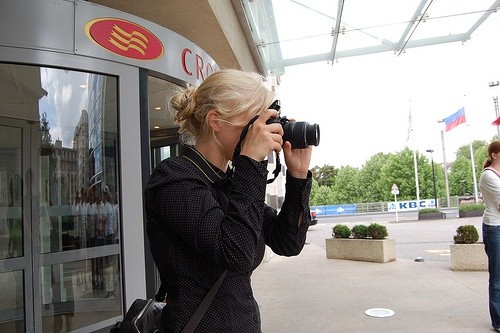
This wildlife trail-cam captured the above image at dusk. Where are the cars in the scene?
[310,210,318,225]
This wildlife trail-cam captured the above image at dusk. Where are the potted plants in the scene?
[459,203,486,218]
[325,223,397,263]
[450,225,489,272]
[418,208,443,220]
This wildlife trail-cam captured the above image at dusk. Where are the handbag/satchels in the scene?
[110,299,166,333]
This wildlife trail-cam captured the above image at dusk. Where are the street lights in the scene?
[426,149,437,208]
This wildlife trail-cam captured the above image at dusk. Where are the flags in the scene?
[490,117,500,126]
[441,106,467,132]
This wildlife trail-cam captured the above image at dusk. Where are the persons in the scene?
[479,140,500,331]
[143,70,312,332]
[67,184,117,298]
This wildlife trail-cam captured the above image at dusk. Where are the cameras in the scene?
[266,105,320,149]
